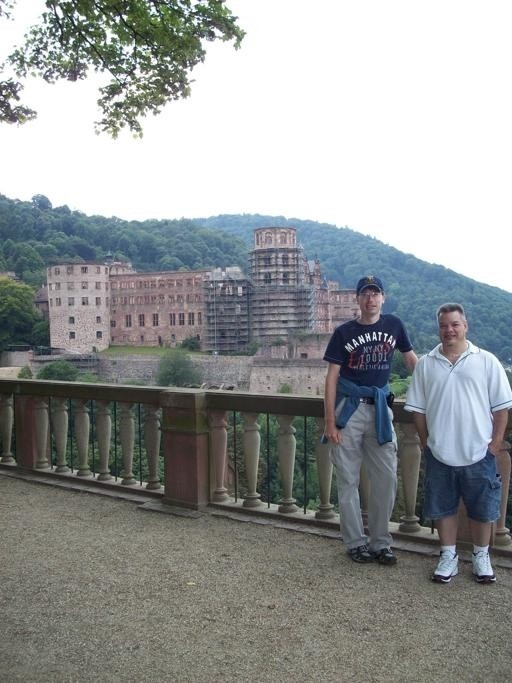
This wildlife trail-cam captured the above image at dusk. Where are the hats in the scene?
[357,277,384,296]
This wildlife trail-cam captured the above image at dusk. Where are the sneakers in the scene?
[350,545,396,565]
[432,551,459,583]
[472,551,496,583]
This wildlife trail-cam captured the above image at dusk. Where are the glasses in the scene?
[359,292,382,297]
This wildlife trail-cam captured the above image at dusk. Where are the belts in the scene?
[358,397,376,405]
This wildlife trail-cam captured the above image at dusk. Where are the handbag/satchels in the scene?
[386,390,396,408]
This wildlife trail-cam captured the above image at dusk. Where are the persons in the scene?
[322,274,420,567]
[401,301,512,586]
